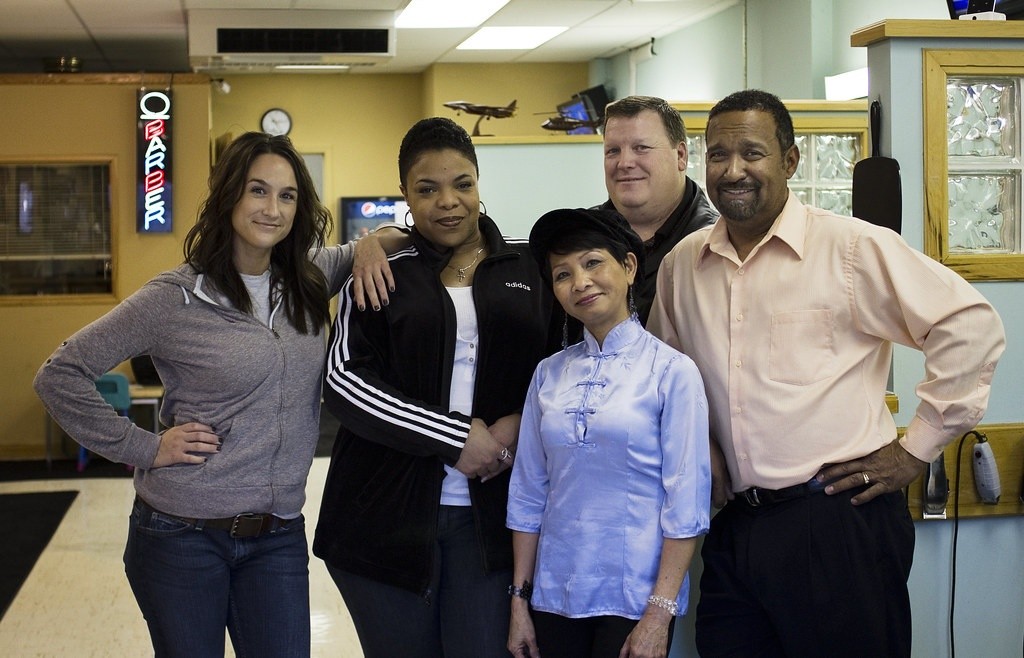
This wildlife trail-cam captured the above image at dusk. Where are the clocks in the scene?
[260,107,292,135]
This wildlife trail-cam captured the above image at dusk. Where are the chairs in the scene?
[76,373,134,472]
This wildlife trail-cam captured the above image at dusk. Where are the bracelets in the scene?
[508,580,533,601]
[647,594,679,616]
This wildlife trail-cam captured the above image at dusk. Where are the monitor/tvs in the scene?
[557,96,597,136]
[340,196,415,245]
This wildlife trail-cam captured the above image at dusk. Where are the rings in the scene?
[497,447,513,464]
[862,472,871,484]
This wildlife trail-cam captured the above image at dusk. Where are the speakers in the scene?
[572,84,611,126]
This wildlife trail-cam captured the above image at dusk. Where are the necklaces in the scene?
[447,236,486,282]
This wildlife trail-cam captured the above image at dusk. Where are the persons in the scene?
[644,89,1007,658]
[505,208,712,658]
[546,95,723,358]
[32,131,413,658]
[311,116,555,658]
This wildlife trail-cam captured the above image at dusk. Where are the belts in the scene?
[733,474,850,508]
[139,493,290,539]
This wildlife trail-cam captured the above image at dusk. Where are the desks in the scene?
[46,384,164,472]
